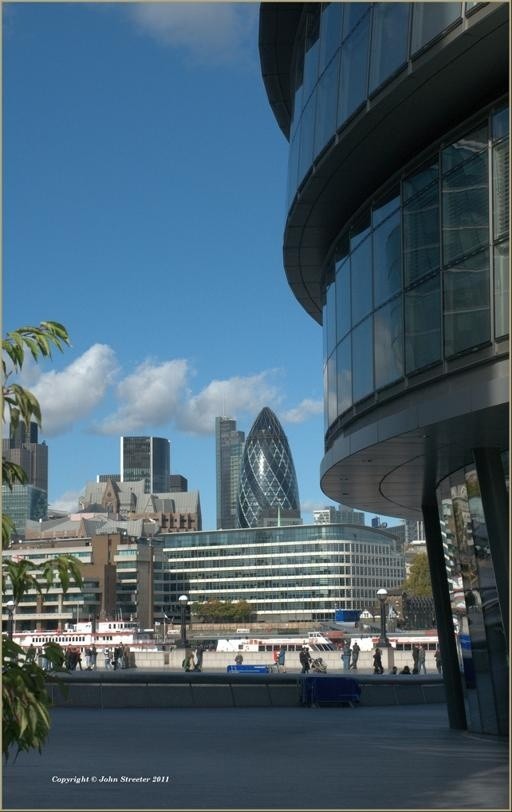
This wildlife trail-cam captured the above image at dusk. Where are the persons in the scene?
[194,641,208,668]
[183,647,197,671]
[234,650,243,665]
[299,641,442,674]
[279,647,285,666]
[272,644,281,664]
[42,641,131,671]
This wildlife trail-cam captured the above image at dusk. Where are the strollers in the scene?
[307,658,328,674]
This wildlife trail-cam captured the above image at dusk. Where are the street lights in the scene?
[178,595,189,648]
[6,600,16,642]
[376,589,391,647]
